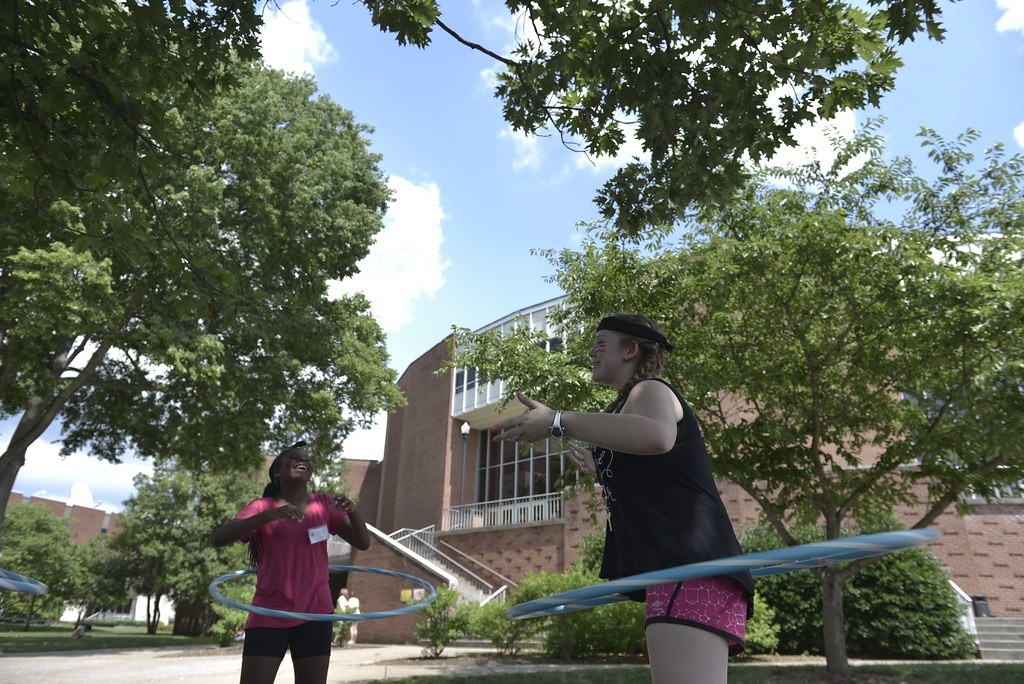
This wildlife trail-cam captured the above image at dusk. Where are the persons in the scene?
[209,440,371,684]
[332,586,362,646]
[71,621,86,641]
[490,312,756,684]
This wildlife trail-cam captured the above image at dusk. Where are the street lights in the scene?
[460,421,472,505]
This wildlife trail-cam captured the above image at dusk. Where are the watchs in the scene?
[549,408,566,440]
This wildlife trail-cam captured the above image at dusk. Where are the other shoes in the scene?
[347,640,356,645]
